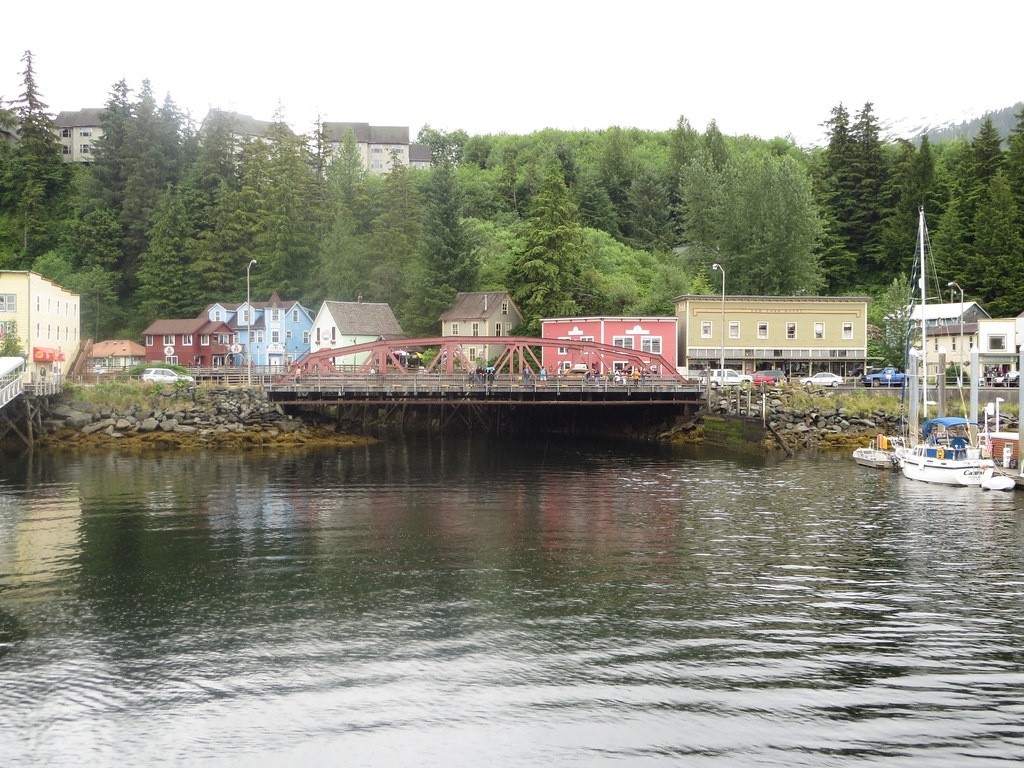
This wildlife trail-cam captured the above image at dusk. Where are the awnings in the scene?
[33,346,65,362]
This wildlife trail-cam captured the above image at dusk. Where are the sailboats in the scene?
[893,202,995,490]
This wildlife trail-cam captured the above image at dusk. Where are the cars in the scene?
[748,375,776,386]
[849,368,864,377]
[1005,370,1020,387]
[798,371,845,388]
[754,370,789,381]
[791,371,806,376]
[141,367,196,383]
[993,373,1008,386]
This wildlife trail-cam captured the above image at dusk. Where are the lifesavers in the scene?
[936,448,945,459]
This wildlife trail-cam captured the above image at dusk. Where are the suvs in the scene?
[698,368,753,389]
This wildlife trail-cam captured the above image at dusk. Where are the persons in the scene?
[615,370,628,384]
[524,365,531,380]
[482,368,495,386]
[631,369,640,385]
[540,366,547,385]
[468,368,474,384]
[583,371,590,384]
[594,368,600,387]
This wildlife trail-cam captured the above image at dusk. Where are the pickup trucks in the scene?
[863,367,910,388]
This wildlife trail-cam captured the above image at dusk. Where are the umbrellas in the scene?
[393,349,409,362]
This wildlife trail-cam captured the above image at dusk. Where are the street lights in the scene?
[247,259,259,388]
[947,282,965,387]
[712,263,725,388]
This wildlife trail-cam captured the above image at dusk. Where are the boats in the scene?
[853,448,898,468]
